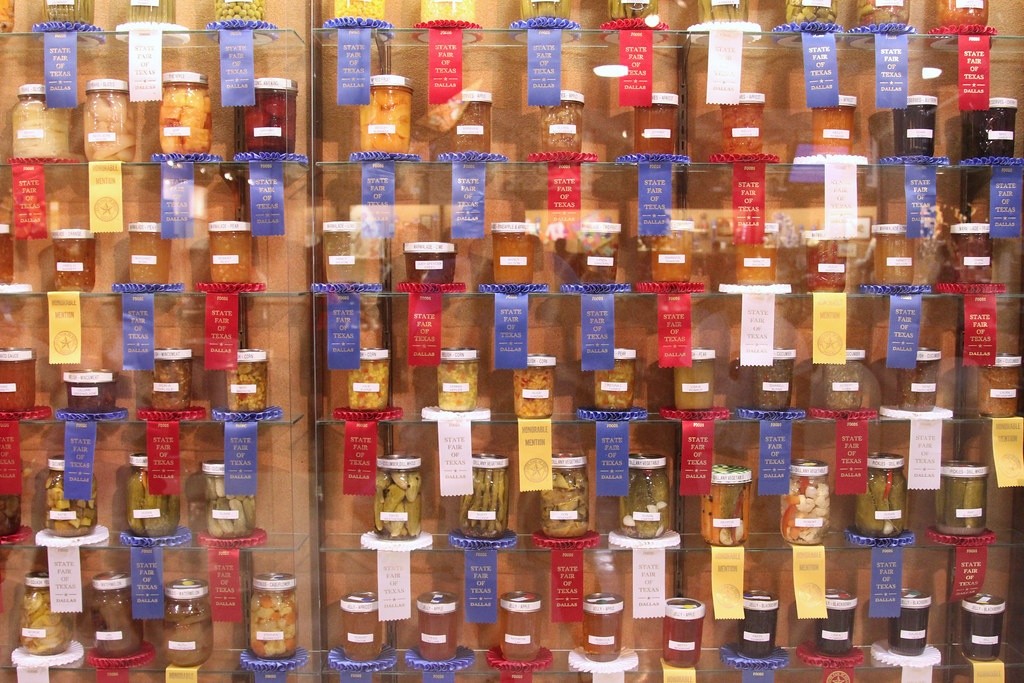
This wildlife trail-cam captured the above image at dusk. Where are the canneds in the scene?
[0,0,1024,683]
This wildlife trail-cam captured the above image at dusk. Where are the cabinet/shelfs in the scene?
[0,34,1024,677]
